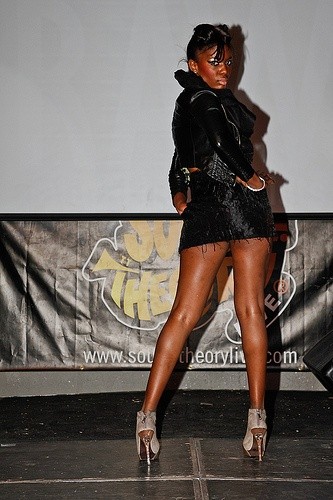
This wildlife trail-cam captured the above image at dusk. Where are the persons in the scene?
[136,22,279,464]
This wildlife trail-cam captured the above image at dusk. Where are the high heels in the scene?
[134,411,162,465]
[242,410,267,461]
